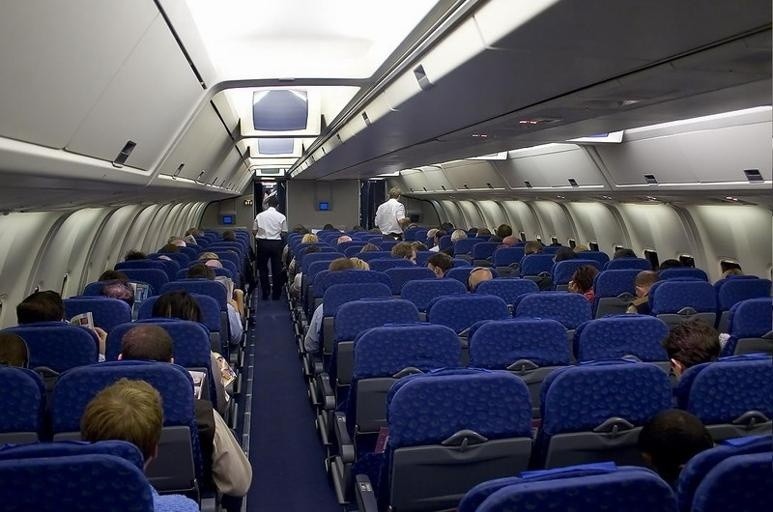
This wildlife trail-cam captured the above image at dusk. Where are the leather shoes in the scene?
[272,291,281,300]
[262,291,271,300]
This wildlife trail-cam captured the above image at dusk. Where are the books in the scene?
[214,276,234,302]
[64,311,94,329]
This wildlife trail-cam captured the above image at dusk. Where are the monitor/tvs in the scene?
[319,203,329,210]
[251,138,303,159]
[223,216,230,223]
[255,169,286,177]
[240,86,321,137]
[410,215,419,222]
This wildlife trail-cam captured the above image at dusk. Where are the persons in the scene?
[82,379,200,511]
[426,228,440,252]
[475,228,491,239]
[626,270,662,314]
[186,264,243,344]
[451,230,467,242]
[468,267,492,293]
[664,320,722,380]
[613,248,636,260]
[199,252,223,268]
[660,259,680,271]
[304,302,324,353]
[252,197,287,300]
[489,223,512,242]
[223,231,236,242]
[337,235,352,244]
[17,290,107,364]
[281,244,287,270]
[162,245,180,254]
[360,243,381,252]
[169,236,187,247]
[375,187,410,241]
[289,234,319,273]
[323,224,332,230]
[185,227,199,240]
[556,247,576,261]
[126,249,147,260]
[574,243,588,253]
[637,408,715,488]
[503,236,520,249]
[391,242,417,265]
[426,253,453,279]
[161,298,236,403]
[117,324,251,496]
[97,284,135,309]
[525,242,542,256]
[97,269,131,285]
[288,246,319,302]
[721,269,743,279]
[569,266,598,301]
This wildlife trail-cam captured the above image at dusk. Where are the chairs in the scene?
[344,367,533,512]
[61,297,132,335]
[79,280,154,314]
[329,323,465,507]
[467,319,575,433]
[425,294,510,350]
[721,299,773,357]
[528,357,676,471]
[590,270,658,316]
[514,292,593,359]
[52,360,213,492]
[103,319,238,439]
[715,277,772,313]
[303,281,393,377]
[474,279,540,305]
[402,279,469,314]
[159,279,245,368]
[551,258,601,291]
[0,365,47,443]
[278,220,526,284]
[309,300,419,407]
[539,245,573,256]
[135,294,244,368]
[0,322,100,388]
[605,258,653,270]
[660,267,710,284]
[0,441,215,512]
[459,462,678,512]
[520,254,558,283]
[676,433,773,512]
[115,225,255,290]
[675,353,773,446]
[112,270,170,293]
[574,314,669,378]
[646,278,719,327]
